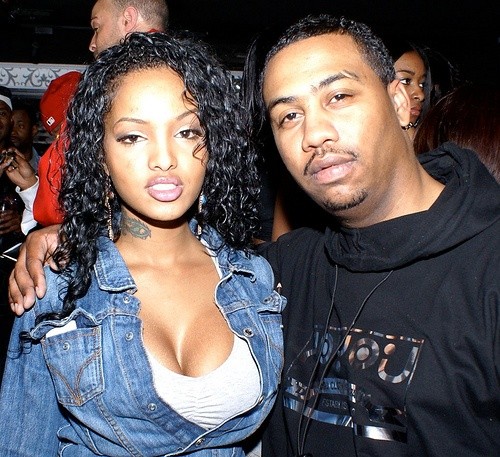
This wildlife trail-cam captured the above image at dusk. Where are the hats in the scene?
[40,71,81,138]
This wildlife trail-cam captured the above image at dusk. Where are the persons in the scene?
[0,30,289,457]
[386,40,500,186]
[7,13,500,457]
[88,0,169,60]
[0,71,84,237]
